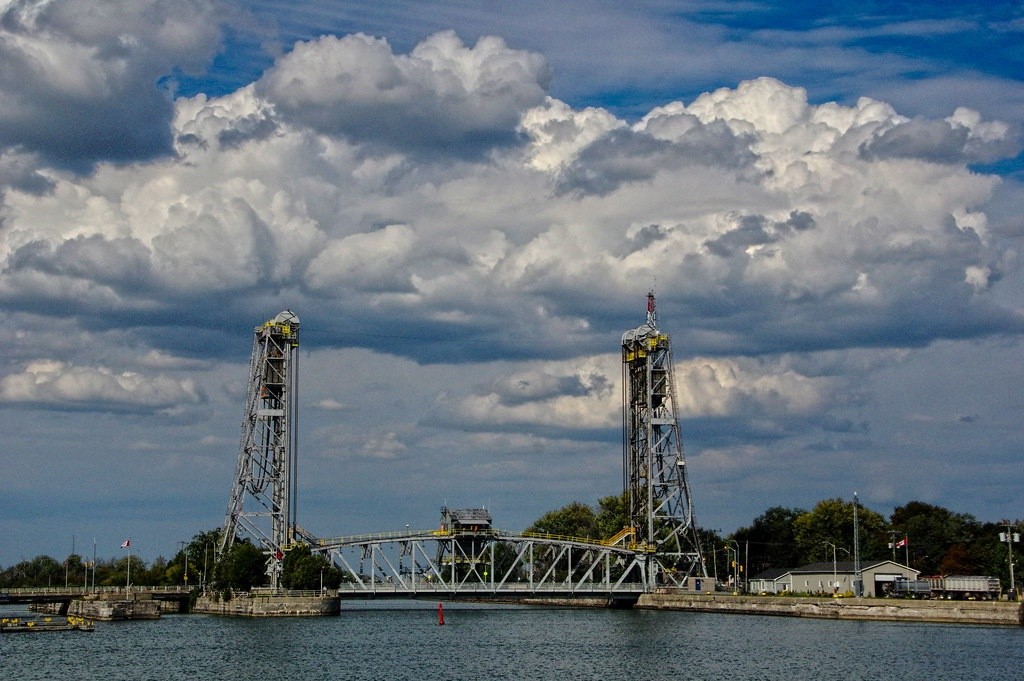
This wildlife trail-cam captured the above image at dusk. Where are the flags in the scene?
[121,540,130,546]
[896,539,905,548]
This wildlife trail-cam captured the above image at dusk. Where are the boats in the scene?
[0,615,95,633]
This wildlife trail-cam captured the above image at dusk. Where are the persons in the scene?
[1008,587,1017,600]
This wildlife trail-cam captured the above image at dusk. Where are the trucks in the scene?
[884,577,931,600]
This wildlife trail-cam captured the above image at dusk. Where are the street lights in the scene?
[724,540,738,594]
[821,540,849,598]
